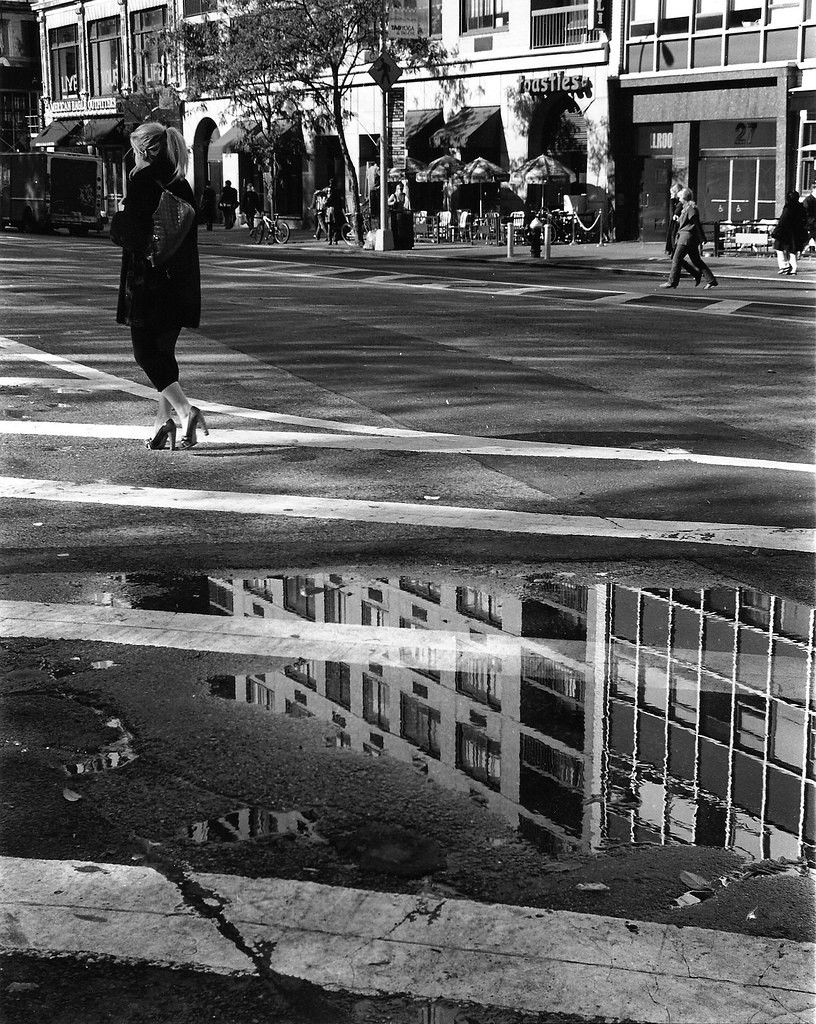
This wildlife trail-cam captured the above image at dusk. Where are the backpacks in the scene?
[316,192,327,211]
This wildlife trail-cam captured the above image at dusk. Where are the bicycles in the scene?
[254,209,289,244]
[524,209,582,245]
[341,213,378,248]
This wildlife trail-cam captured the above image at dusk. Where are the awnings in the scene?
[82,117,124,141]
[406,106,500,148]
[270,120,297,134]
[213,119,262,148]
[29,120,83,147]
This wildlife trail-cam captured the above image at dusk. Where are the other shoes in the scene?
[314,235,319,240]
[250,229,254,236]
[695,273,702,286]
[334,242,337,245]
[660,283,676,288]
[328,242,332,245]
[778,267,791,274]
[704,281,718,290]
[785,272,797,276]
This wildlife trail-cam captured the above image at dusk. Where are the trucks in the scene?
[0,150,101,237]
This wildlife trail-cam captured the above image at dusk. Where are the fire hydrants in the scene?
[528,218,542,257]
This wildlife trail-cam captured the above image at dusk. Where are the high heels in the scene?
[179,406,209,450]
[146,419,176,451]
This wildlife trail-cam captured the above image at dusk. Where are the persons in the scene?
[324,179,341,245]
[307,184,328,241]
[388,181,411,210]
[240,183,258,236]
[660,183,718,290]
[220,180,237,230]
[201,180,216,232]
[110,123,209,451]
[797,186,816,260]
[771,190,803,276]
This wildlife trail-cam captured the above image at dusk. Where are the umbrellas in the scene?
[416,155,465,211]
[509,155,576,215]
[449,157,510,238]
[406,157,426,175]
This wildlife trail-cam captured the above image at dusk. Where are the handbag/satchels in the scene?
[134,171,196,268]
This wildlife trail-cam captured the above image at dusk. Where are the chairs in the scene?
[434,210,451,240]
[505,211,525,246]
[447,211,471,242]
[484,213,504,244]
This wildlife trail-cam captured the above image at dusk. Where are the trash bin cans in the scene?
[391,209,414,250]
